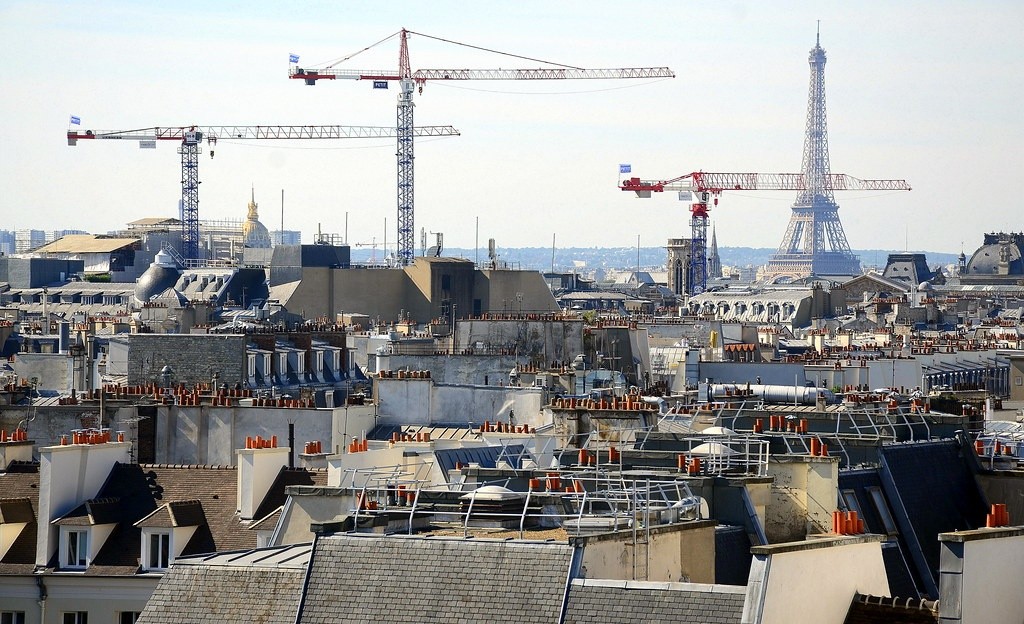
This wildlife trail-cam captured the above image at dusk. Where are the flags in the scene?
[621,165,632,173]
[71,116,80,124]
[290,54,299,63]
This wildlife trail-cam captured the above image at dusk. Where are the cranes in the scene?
[68,125,463,267]
[615,169,912,296]
[287,28,674,259]
